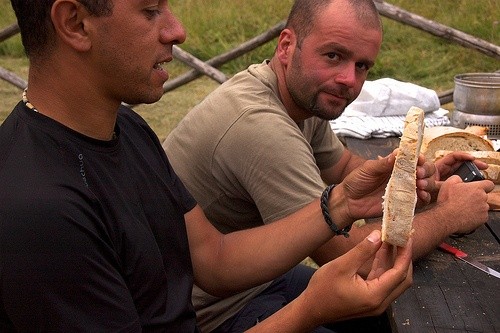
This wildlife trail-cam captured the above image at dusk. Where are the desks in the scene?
[344,118,500,333]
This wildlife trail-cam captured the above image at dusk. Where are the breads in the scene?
[381,106,500,248]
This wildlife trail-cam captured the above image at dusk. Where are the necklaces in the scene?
[21,88,117,141]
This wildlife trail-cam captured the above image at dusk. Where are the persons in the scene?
[0,0,438,332]
[163,0,496,331]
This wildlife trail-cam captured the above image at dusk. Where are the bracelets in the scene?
[319,184,353,239]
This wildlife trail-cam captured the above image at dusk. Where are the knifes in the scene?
[438,240,500,283]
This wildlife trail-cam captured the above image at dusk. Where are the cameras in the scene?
[453,159,485,184]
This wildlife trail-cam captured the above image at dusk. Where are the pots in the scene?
[453,73,500,117]
